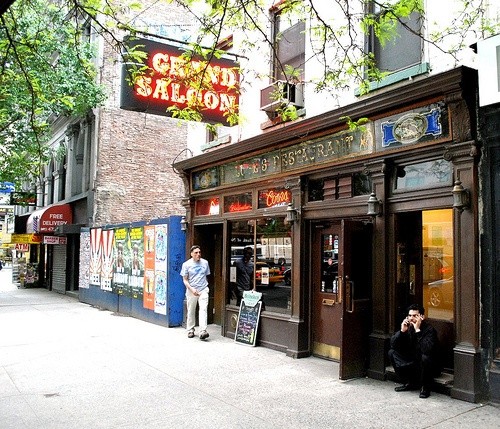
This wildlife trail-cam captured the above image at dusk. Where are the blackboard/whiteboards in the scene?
[234,299,262,348]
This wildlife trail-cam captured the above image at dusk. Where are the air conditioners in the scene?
[260,81,303,112]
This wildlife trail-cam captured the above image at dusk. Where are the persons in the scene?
[388,305,445,399]
[115,243,144,277]
[180,246,210,340]
[233,247,255,307]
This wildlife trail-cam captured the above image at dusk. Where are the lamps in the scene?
[367,191,383,219]
[451,179,469,215]
[180,216,188,233]
[286,203,299,226]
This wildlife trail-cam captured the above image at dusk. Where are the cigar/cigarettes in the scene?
[420,316,424,320]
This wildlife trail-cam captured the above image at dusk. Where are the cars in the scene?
[426,276,453,309]
[229,244,338,293]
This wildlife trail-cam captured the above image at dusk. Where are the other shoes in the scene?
[200,332,209,339]
[419,388,432,398]
[395,386,421,391]
[188,332,194,338]
